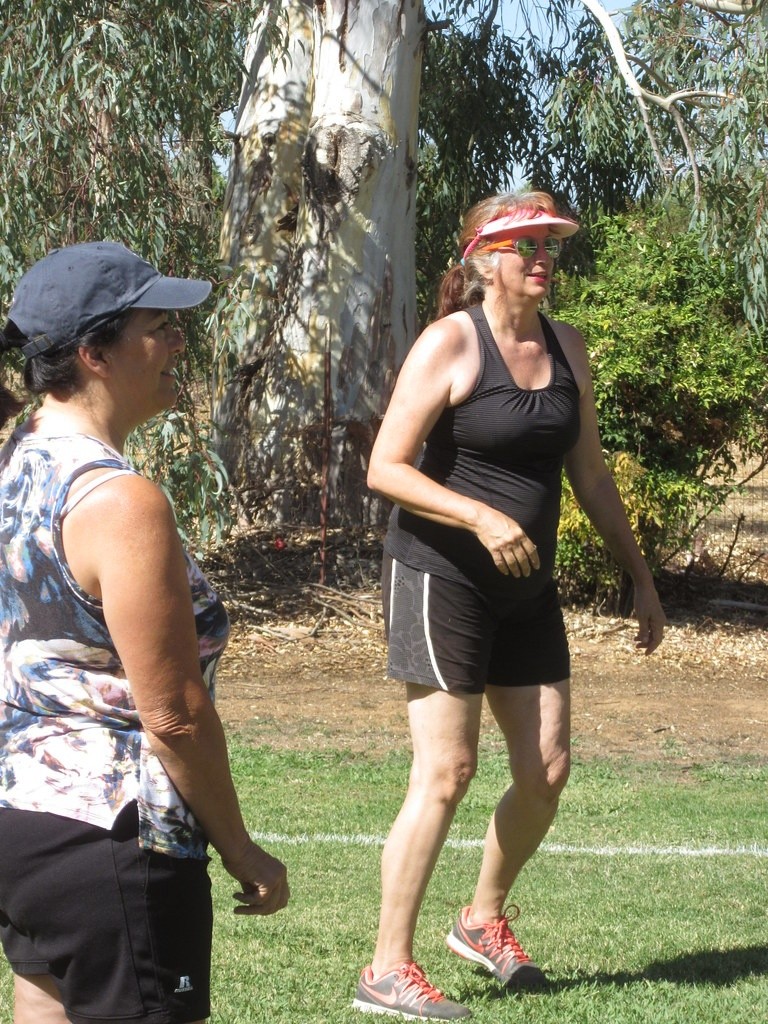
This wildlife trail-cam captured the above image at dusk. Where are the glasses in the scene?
[472,234,566,260]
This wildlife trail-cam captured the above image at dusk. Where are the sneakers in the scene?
[445,904,548,990]
[352,964,473,1023]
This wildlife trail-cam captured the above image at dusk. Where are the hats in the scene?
[462,209,580,261]
[8,241,212,361]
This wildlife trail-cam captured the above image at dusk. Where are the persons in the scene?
[349,192,664,1024]
[0,240,290,1024]
